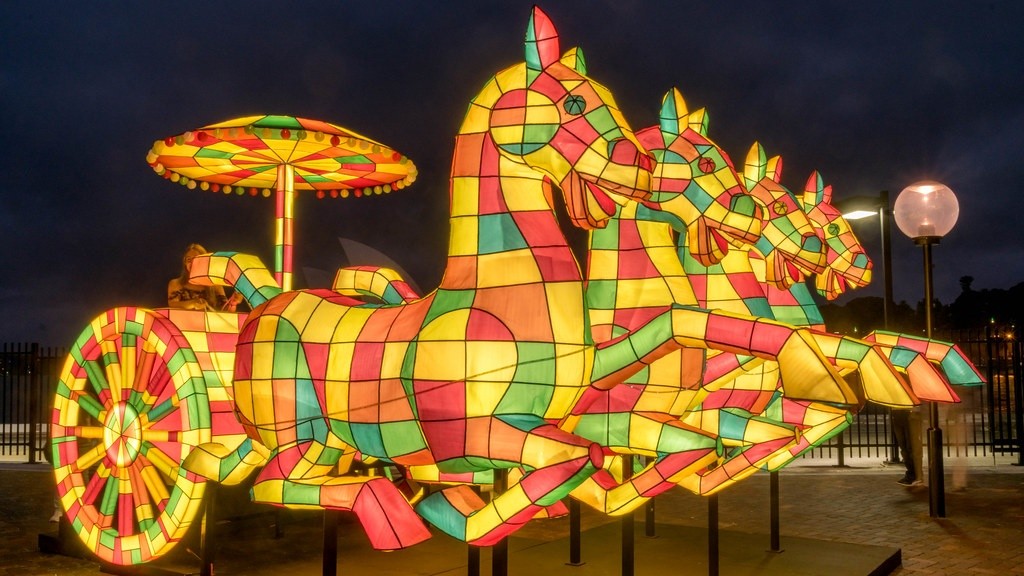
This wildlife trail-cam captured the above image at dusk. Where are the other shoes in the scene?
[896,474,921,487]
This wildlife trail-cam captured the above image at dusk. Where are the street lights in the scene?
[894,181,960,516]
[841,204,899,465]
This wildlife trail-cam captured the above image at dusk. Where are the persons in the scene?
[892,408,922,486]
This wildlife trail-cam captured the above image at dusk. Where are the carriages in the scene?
[48,6,983,567]
